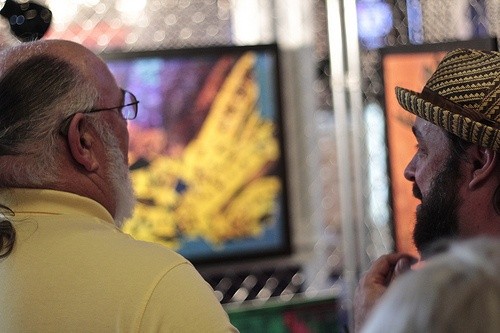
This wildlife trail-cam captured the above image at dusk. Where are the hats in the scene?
[395,45,500,149]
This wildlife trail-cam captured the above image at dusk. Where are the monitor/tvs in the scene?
[91,41,291,267]
[377,36,500,272]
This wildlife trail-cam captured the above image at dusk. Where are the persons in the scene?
[0,37,241,333]
[351,44,500,333]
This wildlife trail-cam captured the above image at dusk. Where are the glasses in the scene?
[58,88,140,133]
